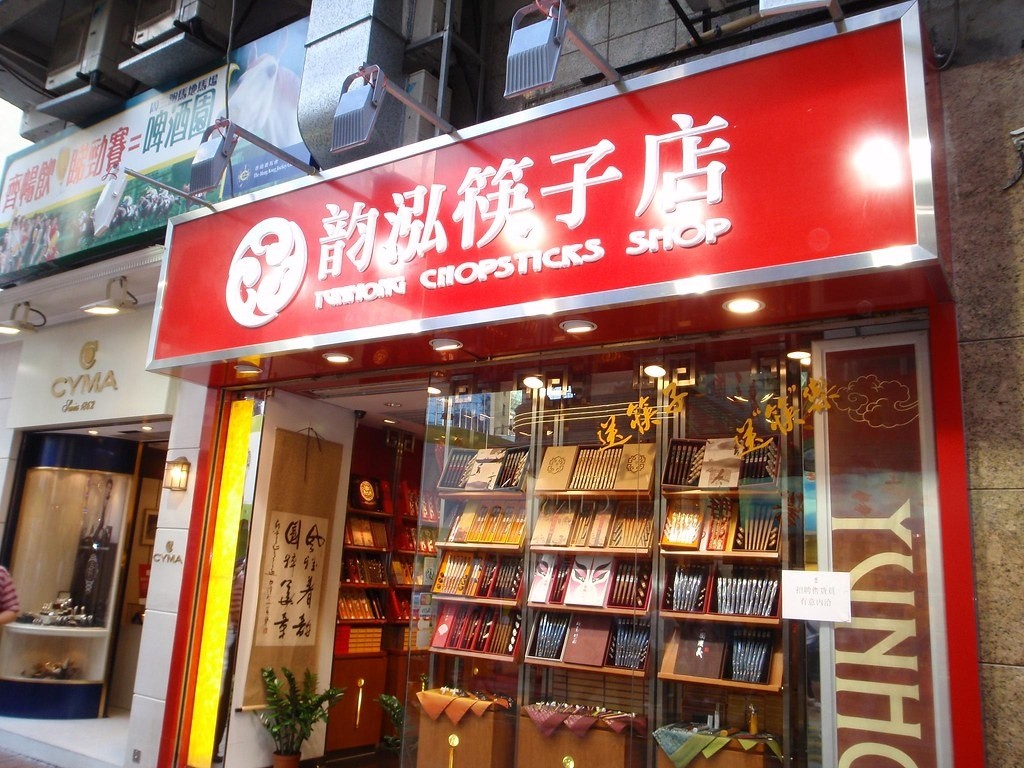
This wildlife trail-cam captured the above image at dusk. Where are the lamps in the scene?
[504,0,621,99]
[161,457,192,491]
[190,116,320,194]
[90,164,213,237]
[79,275,139,316]
[0,302,38,336]
[331,64,457,153]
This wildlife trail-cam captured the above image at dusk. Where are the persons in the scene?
[0,565,21,647]
[0,212,60,273]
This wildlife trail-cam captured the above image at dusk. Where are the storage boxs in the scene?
[336,473,441,652]
[431,433,782,685]
[415,688,518,768]
[518,704,649,768]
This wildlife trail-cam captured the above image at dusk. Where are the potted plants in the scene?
[253,664,347,768]
[378,673,431,768]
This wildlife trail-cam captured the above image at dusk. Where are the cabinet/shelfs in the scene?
[0,618,106,719]
[428,435,800,768]
[323,475,427,757]
[652,720,782,768]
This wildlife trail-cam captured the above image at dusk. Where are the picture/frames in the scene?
[140,508,158,546]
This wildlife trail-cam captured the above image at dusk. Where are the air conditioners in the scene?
[130,0,232,56]
[44,0,137,97]
